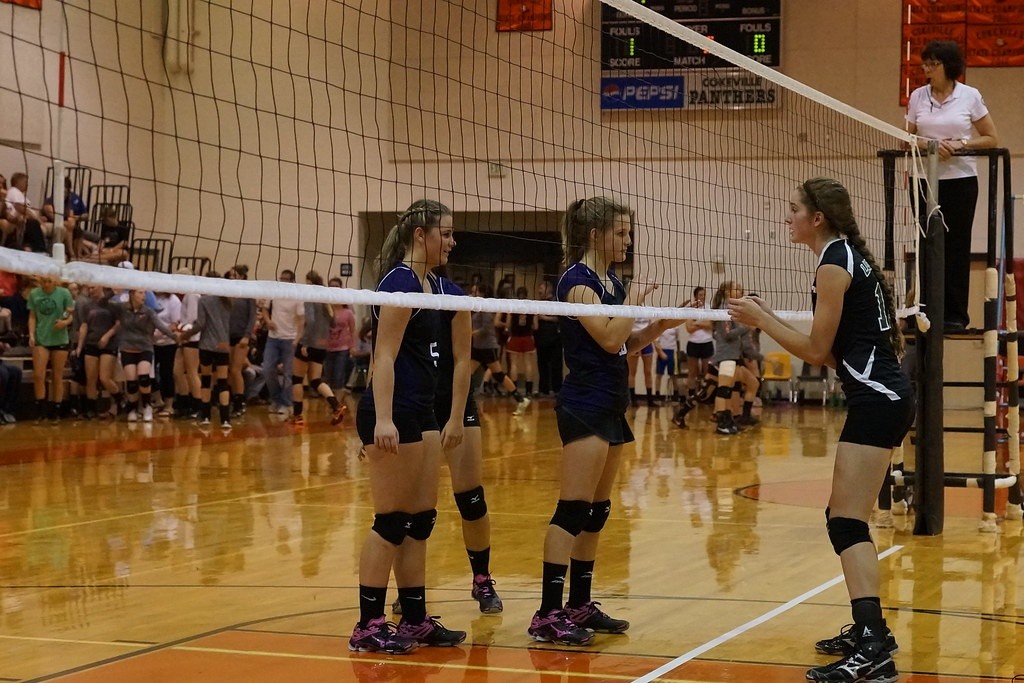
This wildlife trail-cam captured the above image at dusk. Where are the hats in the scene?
[119,262,134,270]
[178,266,194,276]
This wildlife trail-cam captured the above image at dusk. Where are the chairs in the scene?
[665,353,688,401]
[762,353,841,408]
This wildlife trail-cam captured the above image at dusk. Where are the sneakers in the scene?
[351,652,429,683]
[396,615,466,647]
[469,611,503,645]
[348,610,420,654]
[28,395,302,439]
[528,607,595,646]
[329,403,348,425]
[513,399,530,414]
[814,619,900,657]
[591,630,630,658]
[415,641,468,673]
[472,569,503,615]
[804,641,898,683]
[565,598,630,632]
[527,641,606,669]
[391,595,401,613]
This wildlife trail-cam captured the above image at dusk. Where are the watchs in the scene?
[958,139,967,148]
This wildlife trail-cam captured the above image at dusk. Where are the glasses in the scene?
[921,61,944,70]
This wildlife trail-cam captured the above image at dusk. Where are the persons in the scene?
[525,190,704,647]
[903,41,998,335]
[627,279,764,435]
[0,164,372,425]
[725,173,917,683]
[347,196,503,654]
[464,278,565,417]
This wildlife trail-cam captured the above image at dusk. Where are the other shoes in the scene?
[647,394,761,440]
[945,319,965,331]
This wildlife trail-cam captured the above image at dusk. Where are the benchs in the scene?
[0,354,74,382]
